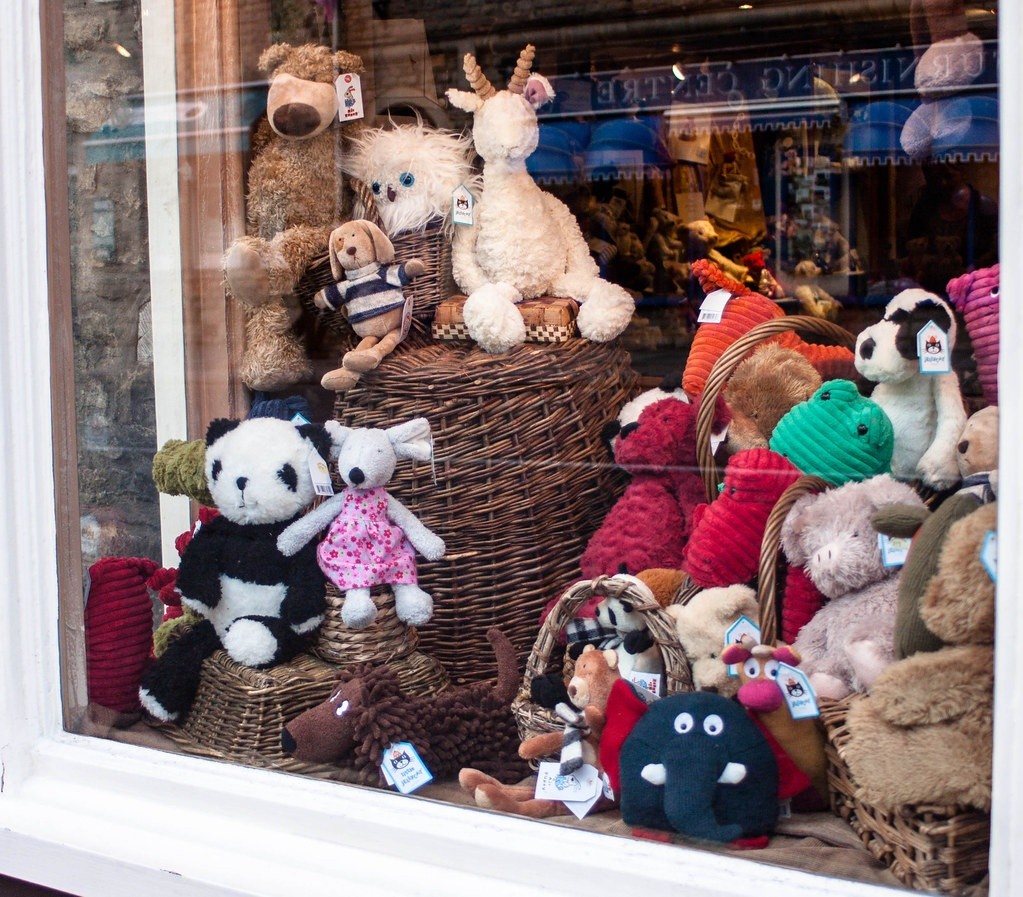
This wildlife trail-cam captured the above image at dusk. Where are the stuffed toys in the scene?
[338,105,474,238]
[135,414,333,728]
[276,416,447,629]
[311,217,426,392]
[459,257,1001,851]
[147,438,257,680]
[542,21,1000,258]
[221,39,368,395]
[443,43,635,355]
[278,626,529,796]
